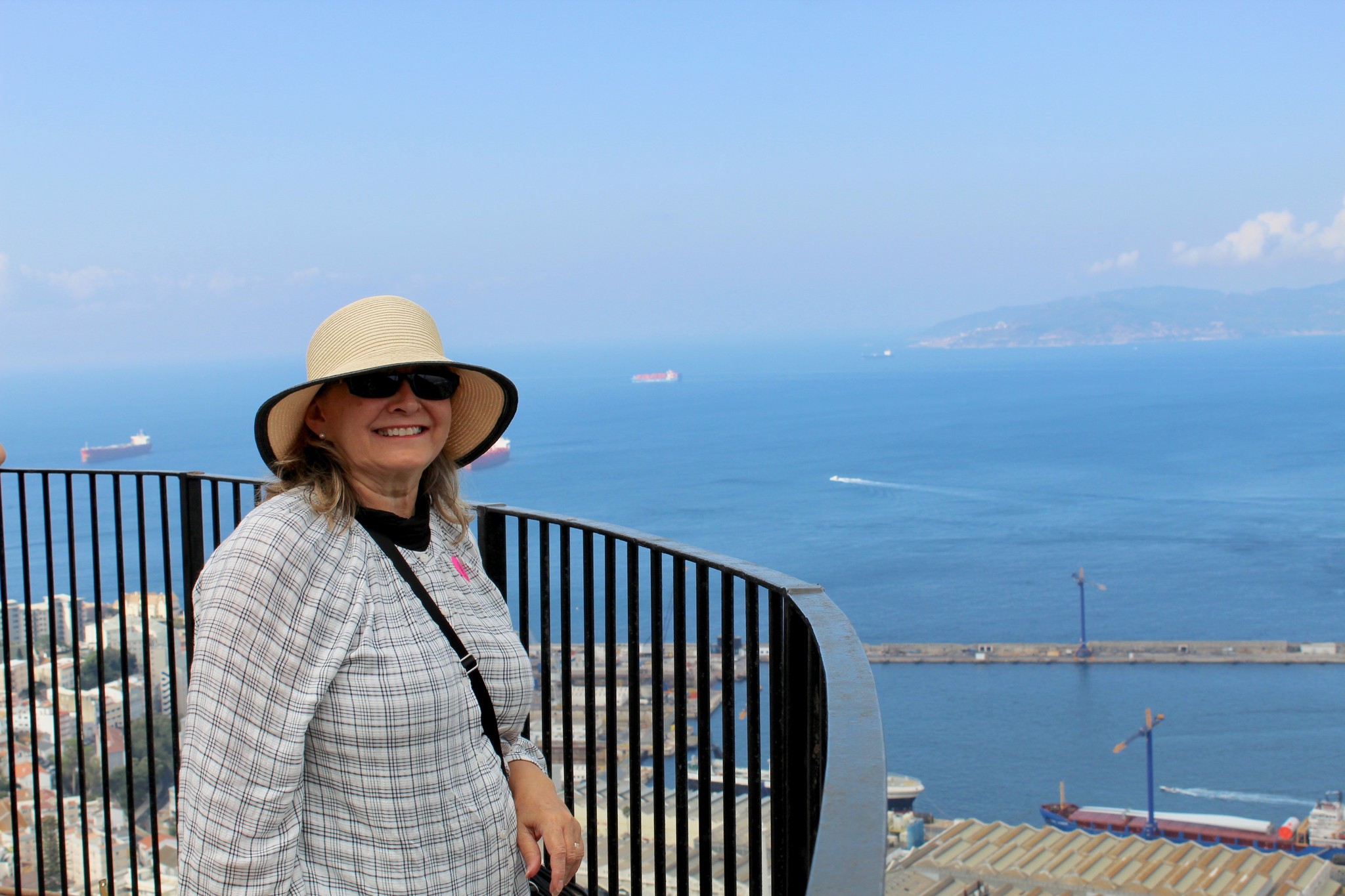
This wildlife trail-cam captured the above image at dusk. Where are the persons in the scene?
[179,293,584,896]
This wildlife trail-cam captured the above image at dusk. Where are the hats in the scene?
[254,296,520,483]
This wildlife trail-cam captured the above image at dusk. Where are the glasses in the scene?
[335,370,461,400]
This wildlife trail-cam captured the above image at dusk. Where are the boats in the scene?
[463,435,512,469]
[82,429,151,465]
[686,754,926,812]
[1040,782,1345,868]
[632,369,681,385]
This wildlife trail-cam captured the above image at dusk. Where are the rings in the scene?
[575,842,580,848]
